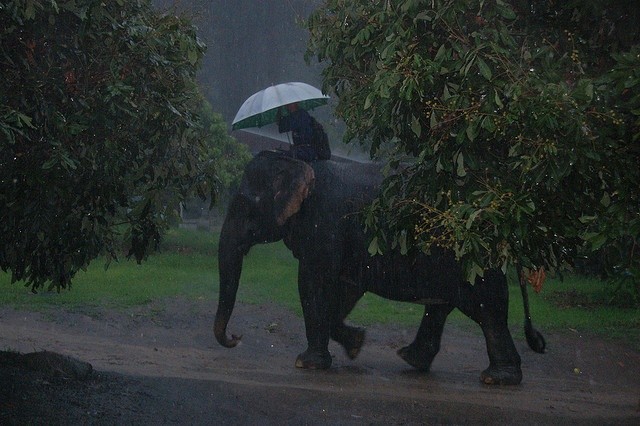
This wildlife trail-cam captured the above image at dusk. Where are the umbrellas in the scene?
[231,81,331,132]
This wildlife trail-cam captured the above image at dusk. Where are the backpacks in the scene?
[308,115,331,159]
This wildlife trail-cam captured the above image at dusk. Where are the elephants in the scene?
[212,147,548,386]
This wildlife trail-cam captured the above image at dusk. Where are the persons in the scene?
[277,103,331,162]
